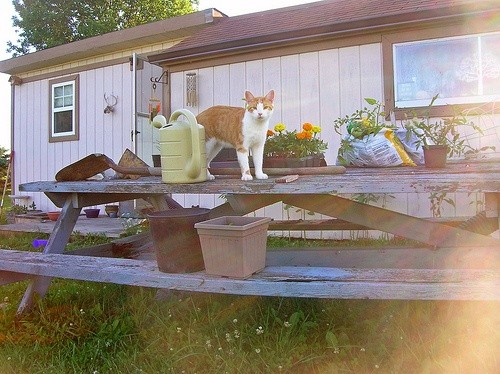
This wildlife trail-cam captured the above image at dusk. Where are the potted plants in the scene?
[400,93,497,168]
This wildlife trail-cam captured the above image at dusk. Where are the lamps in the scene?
[185,72,197,108]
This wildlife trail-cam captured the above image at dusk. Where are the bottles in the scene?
[105,205,119,215]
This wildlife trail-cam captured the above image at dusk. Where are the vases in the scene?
[48,211,61,221]
[194,215,271,279]
[83,209,100,218]
[104,205,119,217]
[248,153,326,168]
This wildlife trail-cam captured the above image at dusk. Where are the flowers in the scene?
[263,121,329,158]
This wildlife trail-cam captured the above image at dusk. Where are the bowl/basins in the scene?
[34,239,47,248]
[109,212,116,217]
[47,211,61,221]
[84,209,100,218]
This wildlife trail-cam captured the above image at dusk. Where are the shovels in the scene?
[55,152,347,182]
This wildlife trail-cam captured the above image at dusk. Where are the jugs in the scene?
[153,108,209,184]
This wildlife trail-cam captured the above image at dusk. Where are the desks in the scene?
[0,154,500,316]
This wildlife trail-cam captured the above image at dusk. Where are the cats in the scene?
[195,90,275,182]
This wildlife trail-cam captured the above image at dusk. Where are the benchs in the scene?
[266,215,475,231]
[0,247,500,303]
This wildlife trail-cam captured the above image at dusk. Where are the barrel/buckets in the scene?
[146,208,213,274]
